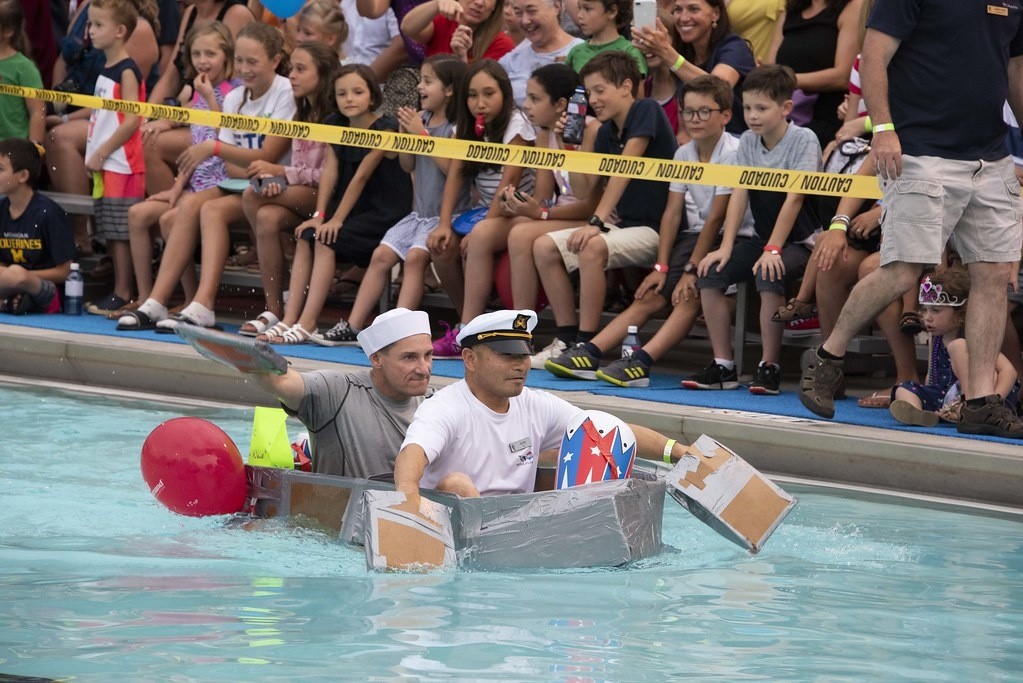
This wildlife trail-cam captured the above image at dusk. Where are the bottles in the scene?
[64,262,84,316]
[563,85,588,145]
[622,326,640,358]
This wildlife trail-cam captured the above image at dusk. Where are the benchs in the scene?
[40,192,938,380]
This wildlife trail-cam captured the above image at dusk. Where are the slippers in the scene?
[173,305,184,315]
[117,307,156,328]
[859,388,893,408]
[5,295,22,314]
[107,302,143,320]
[156,311,223,333]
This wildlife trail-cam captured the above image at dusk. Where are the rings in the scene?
[639,39,643,44]
[857,229,861,233]
[147,128,152,132]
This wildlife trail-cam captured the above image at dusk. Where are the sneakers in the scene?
[749,360,781,394]
[545,339,601,382]
[596,348,651,388]
[680,359,739,389]
[527,338,568,369]
[428,320,467,361]
[311,320,361,345]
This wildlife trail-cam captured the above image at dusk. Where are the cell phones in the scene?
[253,177,288,193]
[502,191,526,203]
[634,0,658,33]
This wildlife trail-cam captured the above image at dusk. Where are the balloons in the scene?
[141,417,248,517]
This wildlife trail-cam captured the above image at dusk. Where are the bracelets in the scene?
[829,224,847,232]
[654,264,668,273]
[831,215,850,224]
[835,218,849,226]
[420,129,429,136]
[313,211,325,219]
[764,245,782,255]
[872,123,894,134]
[62,115,68,123]
[663,439,677,464]
[214,141,220,156]
[669,55,684,71]
[865,116,872,132]
[541,208,549,220]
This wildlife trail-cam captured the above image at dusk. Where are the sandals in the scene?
[254,319,290,341]
[901,311,922,335]
[771,298,817,322]
[798,340,844,418]
[957,393,1023,437]
[269,324,319,343]
[238,311,279,336]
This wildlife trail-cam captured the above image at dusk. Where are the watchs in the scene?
[684,262,698,275]
[589,215,610,233]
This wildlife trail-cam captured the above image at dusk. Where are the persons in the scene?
[801,0,1023,436]
[0,0,1023,407]
[394,309,690,501]
[252,306,440,483]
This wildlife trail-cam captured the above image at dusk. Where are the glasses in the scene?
[679,105,723,121]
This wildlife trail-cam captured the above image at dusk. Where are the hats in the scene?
[456,308,538,355]
[356,307,436,359]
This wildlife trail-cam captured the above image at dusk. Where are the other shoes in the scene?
[84,293,128,315]
[891,399,940,429]
